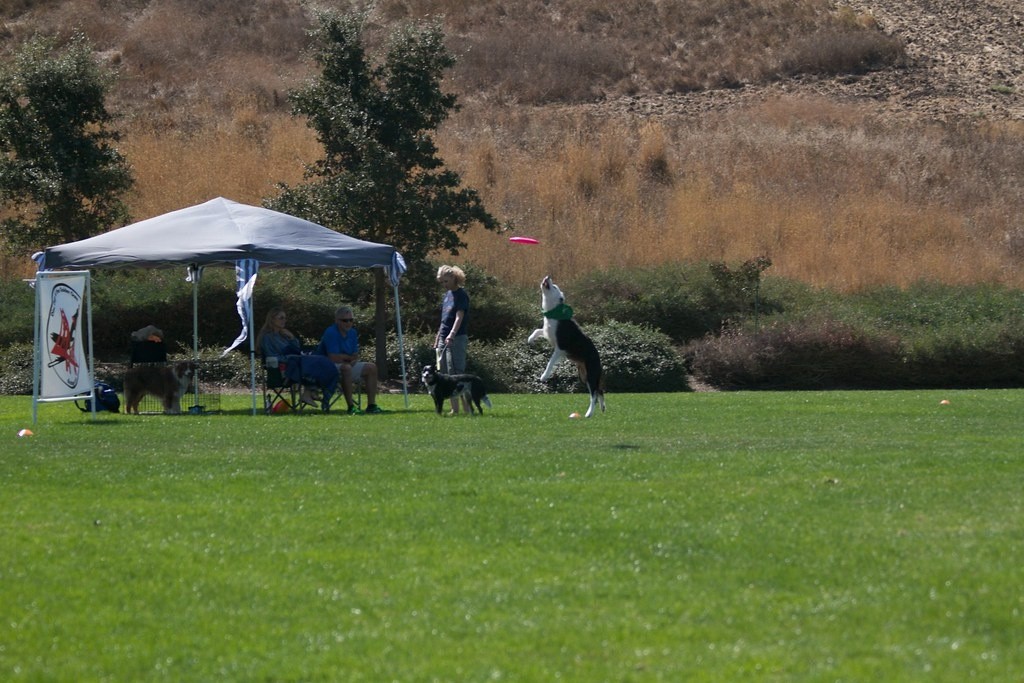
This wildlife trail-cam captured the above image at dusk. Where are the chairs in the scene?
[260,345,361,415]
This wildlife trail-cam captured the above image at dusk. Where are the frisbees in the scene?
[508,236,541,245]
[272,398,291,413]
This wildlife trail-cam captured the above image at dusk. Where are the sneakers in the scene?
[347,405,365,416]
[366,404,385,413]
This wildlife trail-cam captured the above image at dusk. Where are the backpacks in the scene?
[72,382,121,413]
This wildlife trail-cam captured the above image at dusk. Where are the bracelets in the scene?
[450,329,456,335]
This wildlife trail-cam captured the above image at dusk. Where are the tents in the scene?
[31,197,409,424]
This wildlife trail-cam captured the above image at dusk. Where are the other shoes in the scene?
[301,392,322,408]
[447,407,470,415]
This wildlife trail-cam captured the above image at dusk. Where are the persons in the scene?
[433,265,471,416]
[316,306,383,415]
[256,307,323,408]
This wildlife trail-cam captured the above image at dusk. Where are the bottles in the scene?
[265,393,273,415]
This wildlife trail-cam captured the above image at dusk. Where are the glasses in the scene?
[338,318,354,323]
[274,316,287,320]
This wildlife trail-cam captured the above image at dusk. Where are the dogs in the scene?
[123,361,197,414]
[527,275,605,418]
[421,365,492,416]
